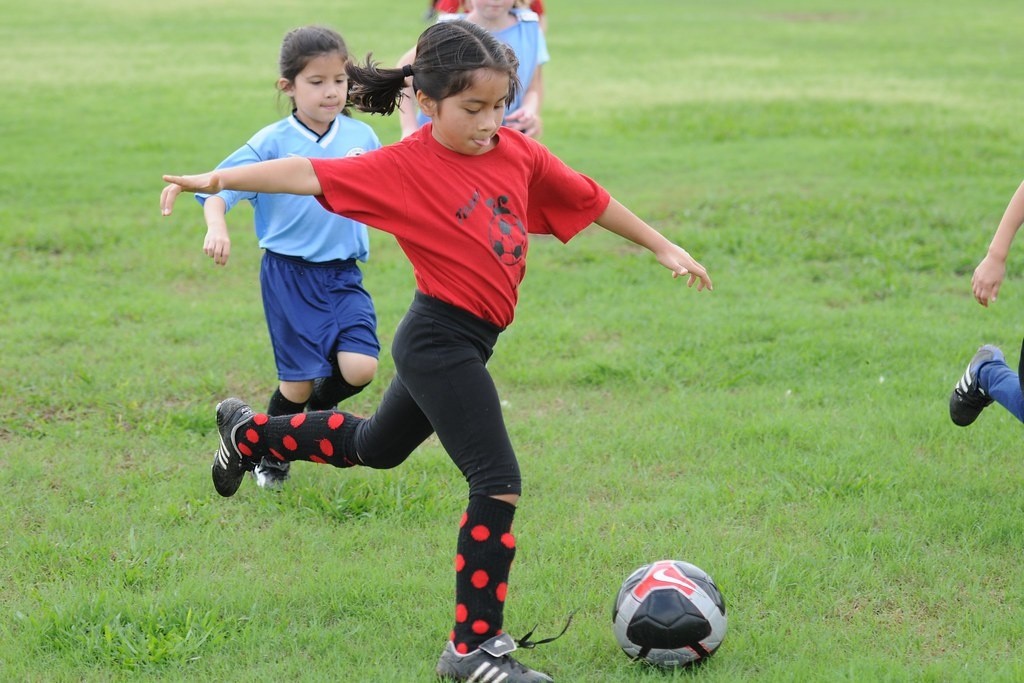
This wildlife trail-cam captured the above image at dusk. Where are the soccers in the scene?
[611,560,729,670]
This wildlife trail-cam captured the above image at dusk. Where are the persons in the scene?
[160,20,713,683]
[394,0,550,142]
[949,178,1024,427]
[194,24,383,492]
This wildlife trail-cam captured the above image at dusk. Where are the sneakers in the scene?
[211,397,256,497]
[949,344,1005,427]
[435,607,582,683]
[249,457,291,488]
